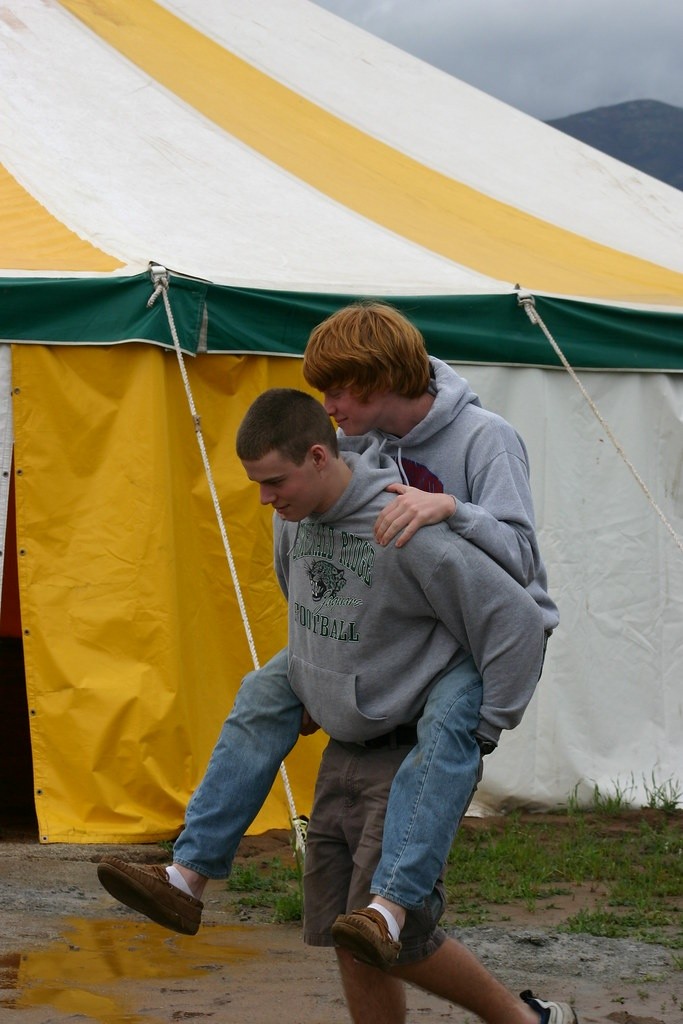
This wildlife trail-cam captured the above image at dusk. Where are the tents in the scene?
[0,2,683,845]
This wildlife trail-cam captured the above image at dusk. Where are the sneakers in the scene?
[520,990,578,1024]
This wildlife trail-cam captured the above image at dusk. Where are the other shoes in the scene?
[97,856,204,936]
[332,907,405,974]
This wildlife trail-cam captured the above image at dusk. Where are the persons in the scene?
[236,388,575,1024]
[96,305,559,973]
